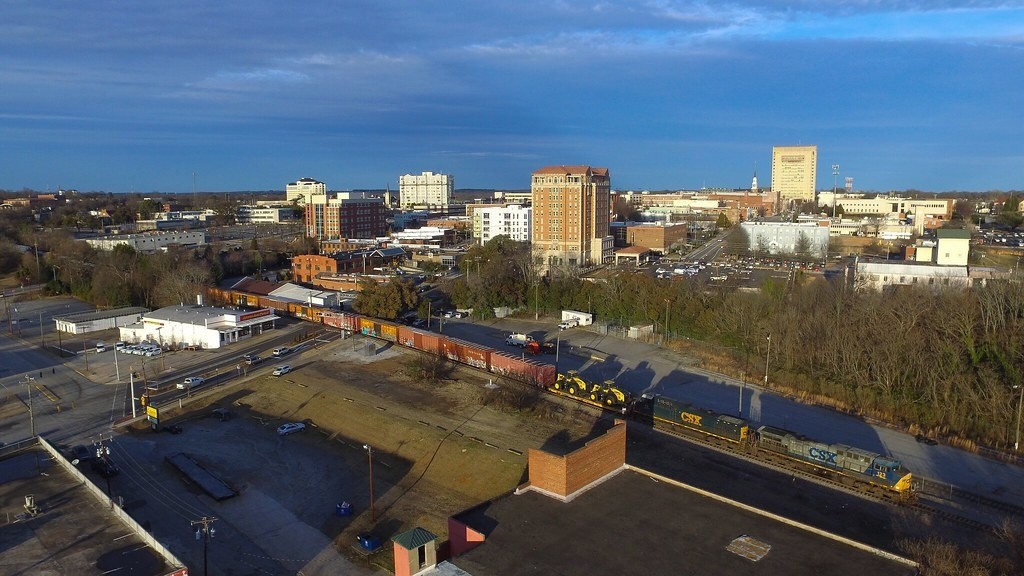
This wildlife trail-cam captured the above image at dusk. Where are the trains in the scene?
[626,390,919,507]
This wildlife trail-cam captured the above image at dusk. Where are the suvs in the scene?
[176,376,205,390]
[272,365,292,377]
[245,356,264,365]
[557,320,578,330]
[504,334,557,357]
[272,347,290,356]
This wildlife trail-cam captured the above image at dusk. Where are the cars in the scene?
[372,263,470,330]
[95,341,162,359]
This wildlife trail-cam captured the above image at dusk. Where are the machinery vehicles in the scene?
[588,380,633,407]
[554,369,591,397]
